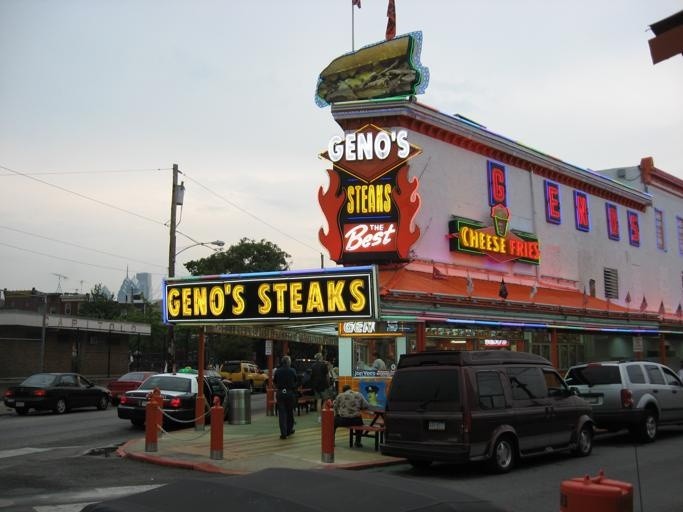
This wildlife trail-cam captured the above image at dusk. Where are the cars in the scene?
[117,370,236,429]
[105,369,166,408]
[3,370,114,415]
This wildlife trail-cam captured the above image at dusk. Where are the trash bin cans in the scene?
[228,388,251,424]
[559,472,633,512]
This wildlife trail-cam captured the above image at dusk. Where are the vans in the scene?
[377,346,600,477]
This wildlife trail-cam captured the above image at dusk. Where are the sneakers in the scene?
[279,428,295,439]
[354,441,363,448]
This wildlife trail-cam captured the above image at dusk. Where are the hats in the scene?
[365,385,379,394]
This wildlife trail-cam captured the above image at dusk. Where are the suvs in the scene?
[562,354,682,446]
[219,359,273,394]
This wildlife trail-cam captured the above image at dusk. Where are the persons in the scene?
[332,384,369,449]
[372,351,386,370]
[307,353,333,423]
[274,356,298,439]
[77,376,82,387]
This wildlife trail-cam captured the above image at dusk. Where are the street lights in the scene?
[167,239,227,371]
